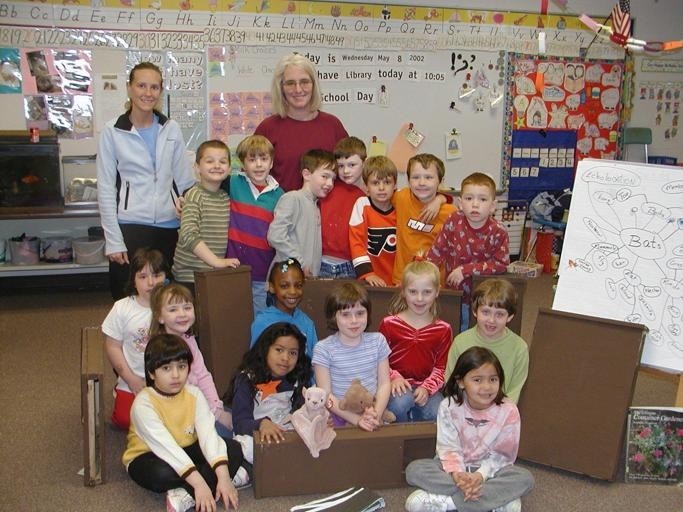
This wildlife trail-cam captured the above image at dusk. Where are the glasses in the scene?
[281,78,313,89]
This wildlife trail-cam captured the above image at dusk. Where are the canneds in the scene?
[30,127,40,143]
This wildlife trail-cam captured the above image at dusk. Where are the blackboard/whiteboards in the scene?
[1,2,637,219]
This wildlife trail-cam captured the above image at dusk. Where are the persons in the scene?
[122,334,254,512]
[443,278,530,406]
[248,257,320,386]
[310,280,393,432]
[218,320,334,445]
[97,55,512,331]
[100,247,169,429]
[378,259,453,423]
[148,281,238,440]
[404,345,535,511]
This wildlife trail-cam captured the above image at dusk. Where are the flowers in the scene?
[630,421,683,482]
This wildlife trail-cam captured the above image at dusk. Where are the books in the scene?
[624,407,683,486]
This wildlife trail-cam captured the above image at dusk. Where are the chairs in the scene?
[621,125,654,164]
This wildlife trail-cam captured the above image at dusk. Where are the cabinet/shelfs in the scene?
[0,199,111,279]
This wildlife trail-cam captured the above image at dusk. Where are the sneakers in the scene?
[164,487,196,512]
[230,465,250,488]
[403,487,448,512]
[489,495,522,512]
